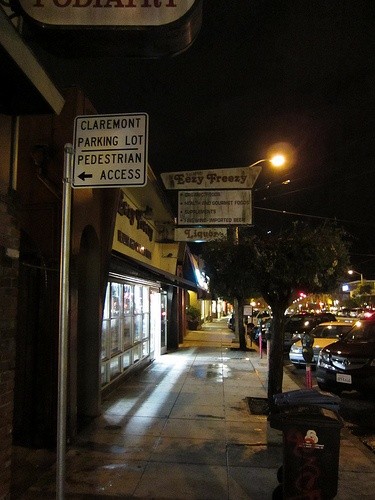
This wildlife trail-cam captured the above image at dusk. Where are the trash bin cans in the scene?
[267,392,344,500]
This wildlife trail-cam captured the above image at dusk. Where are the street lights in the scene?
[347,269,364,288]
[232,154,284,343]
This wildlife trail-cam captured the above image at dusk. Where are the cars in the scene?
[289,319,356,369]
[316,319,375,395]
[228,307,375,349]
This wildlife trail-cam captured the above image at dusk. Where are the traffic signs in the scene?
[71,112,150,188]
